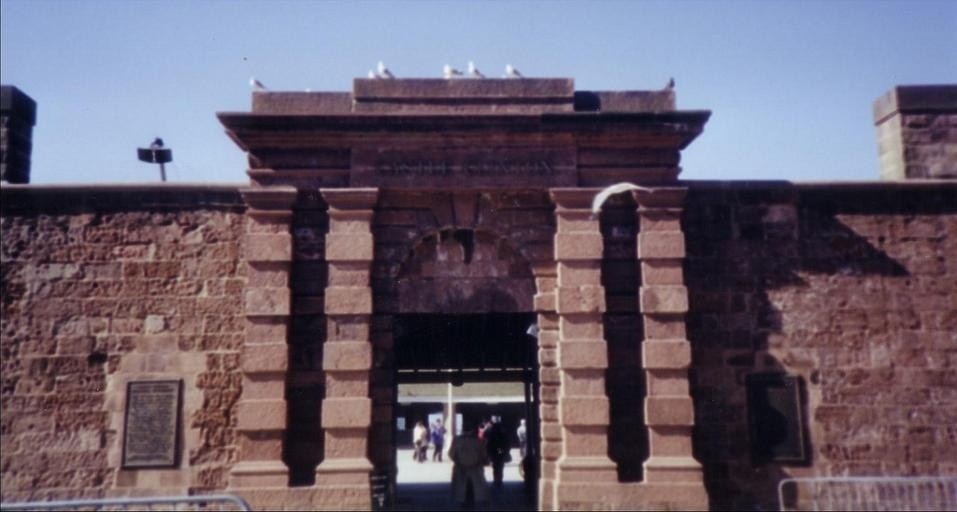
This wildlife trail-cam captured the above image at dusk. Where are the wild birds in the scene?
[249,74,267,92]
[443,61,463,78]
[505,64,522,78]
[367,60,393,78]
[467,60,485,77]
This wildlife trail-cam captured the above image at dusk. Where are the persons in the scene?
[412,415,526,512]
